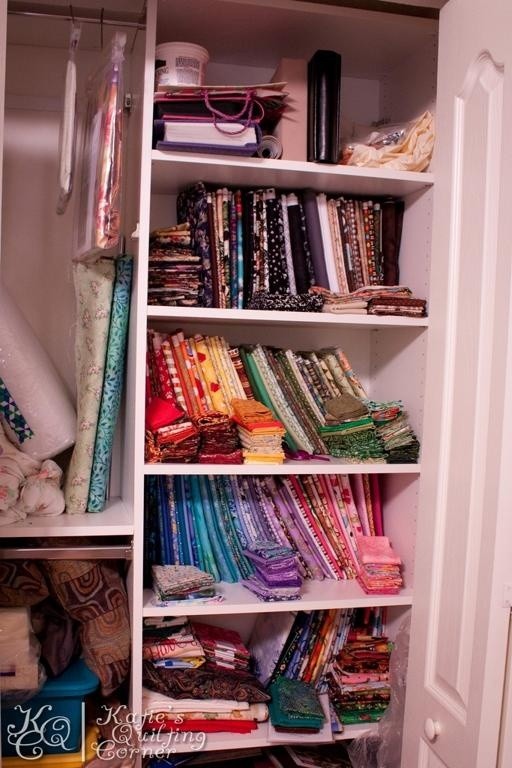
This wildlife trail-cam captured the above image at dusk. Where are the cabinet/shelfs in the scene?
[2,0,435,758]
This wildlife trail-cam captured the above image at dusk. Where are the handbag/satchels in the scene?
[154,82,289,134]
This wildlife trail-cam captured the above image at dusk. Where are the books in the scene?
[153,115,265,158]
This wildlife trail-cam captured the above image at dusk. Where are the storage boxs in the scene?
[2,666,101,766]
